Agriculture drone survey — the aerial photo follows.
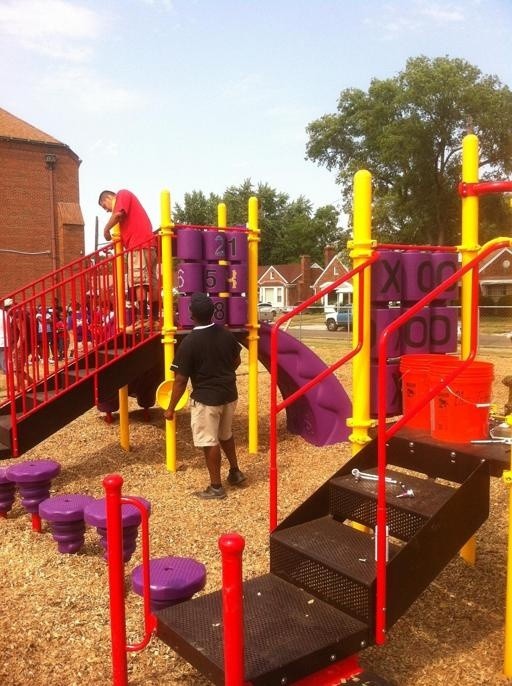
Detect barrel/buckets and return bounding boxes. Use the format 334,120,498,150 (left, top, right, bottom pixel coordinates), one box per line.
399,354,458,431
430,360,492,443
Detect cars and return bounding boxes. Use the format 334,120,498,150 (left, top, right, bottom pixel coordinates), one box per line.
257,303,280,323
324,303,353,332
281,301,315,314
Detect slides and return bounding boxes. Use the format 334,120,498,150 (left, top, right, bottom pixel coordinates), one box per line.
242,320,352,446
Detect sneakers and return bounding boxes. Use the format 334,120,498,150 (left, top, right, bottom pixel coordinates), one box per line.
126,318,159,333
197,486,226,500
227,470,247,487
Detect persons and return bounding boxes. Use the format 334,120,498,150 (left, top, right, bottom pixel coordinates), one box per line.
98,189,159,335
164,292,247,500
0,298,71,375
